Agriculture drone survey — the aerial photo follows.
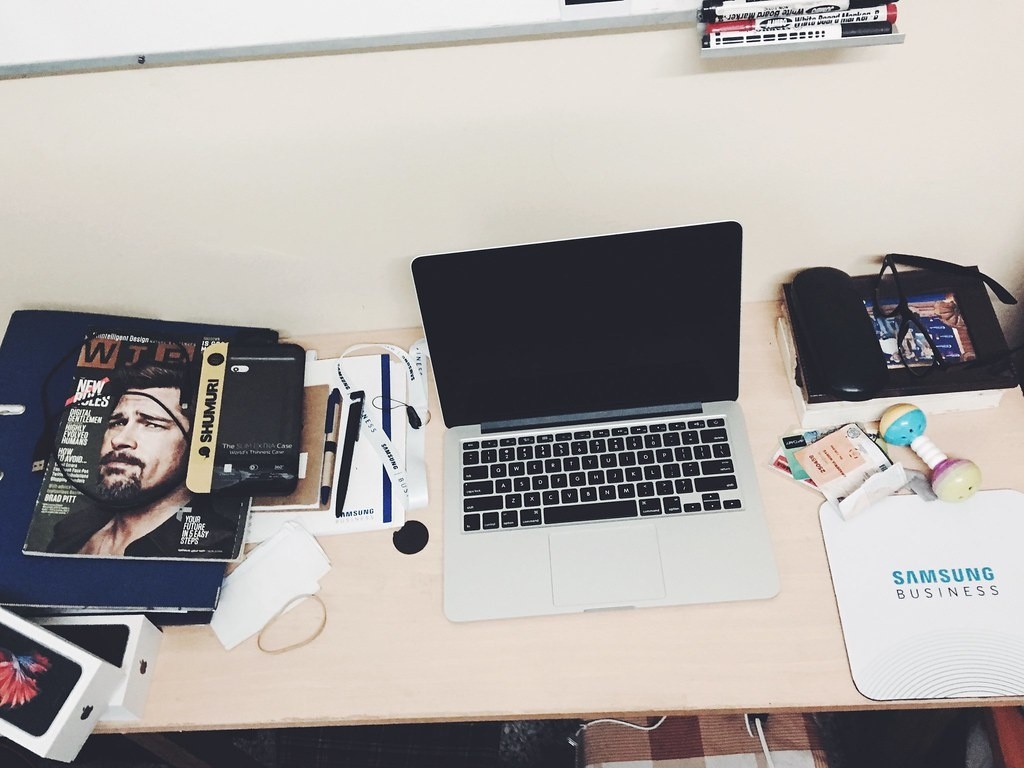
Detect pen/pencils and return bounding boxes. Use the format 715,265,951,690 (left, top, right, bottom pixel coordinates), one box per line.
335,390,364,518
320,388,342,505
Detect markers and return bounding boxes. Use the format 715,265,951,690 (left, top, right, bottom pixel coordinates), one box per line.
696,0,898,48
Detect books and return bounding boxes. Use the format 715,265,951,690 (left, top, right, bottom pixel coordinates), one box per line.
696,0,905,48
22,326,254,561
249,384,333,512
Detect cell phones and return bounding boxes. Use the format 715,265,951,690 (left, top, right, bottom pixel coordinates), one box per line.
186,342,305,496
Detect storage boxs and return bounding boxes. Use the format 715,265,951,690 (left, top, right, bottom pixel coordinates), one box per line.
34,613,165,721
0,605,126,764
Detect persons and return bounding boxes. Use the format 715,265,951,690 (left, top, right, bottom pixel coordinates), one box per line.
46,367,239,557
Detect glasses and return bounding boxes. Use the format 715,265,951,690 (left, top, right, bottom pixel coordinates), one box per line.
875,254,1024,378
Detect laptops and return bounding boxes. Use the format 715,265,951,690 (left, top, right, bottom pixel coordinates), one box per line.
412,221,782,623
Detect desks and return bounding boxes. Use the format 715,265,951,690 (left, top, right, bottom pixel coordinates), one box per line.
0,0,1024,737
